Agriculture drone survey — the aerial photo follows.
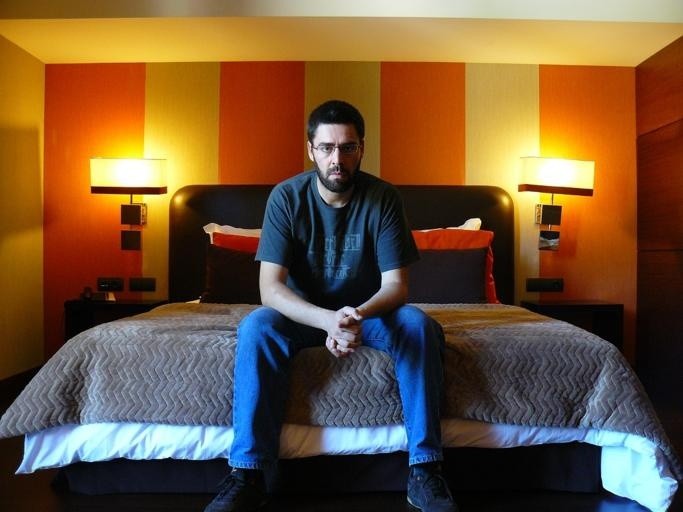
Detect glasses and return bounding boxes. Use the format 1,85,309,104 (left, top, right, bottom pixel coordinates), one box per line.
312,142,360,154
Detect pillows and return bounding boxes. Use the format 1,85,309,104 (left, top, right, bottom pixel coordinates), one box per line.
200,218,499,302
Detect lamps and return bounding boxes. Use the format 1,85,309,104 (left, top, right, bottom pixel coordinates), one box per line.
519,156,593,251
88,160,167,250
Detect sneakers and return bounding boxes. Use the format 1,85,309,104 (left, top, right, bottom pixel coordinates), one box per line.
204,470,267,512
407,471,457,511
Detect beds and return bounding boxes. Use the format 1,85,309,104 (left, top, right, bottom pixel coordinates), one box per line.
0,184,683,511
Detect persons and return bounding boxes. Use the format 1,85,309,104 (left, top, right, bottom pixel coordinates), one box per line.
198,98,460,512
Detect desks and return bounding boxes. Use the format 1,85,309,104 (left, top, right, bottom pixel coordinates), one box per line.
523,300,624,345
63,295,169,336
